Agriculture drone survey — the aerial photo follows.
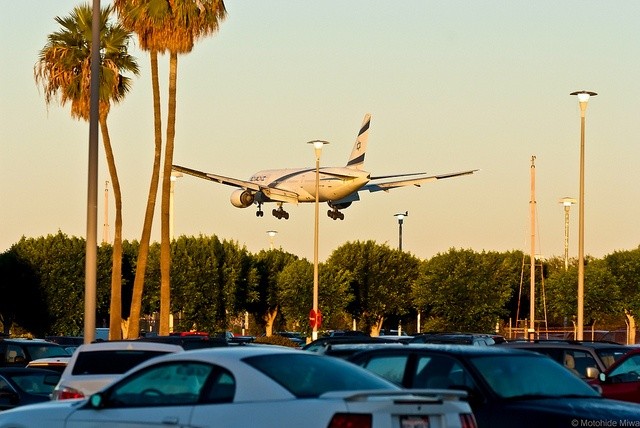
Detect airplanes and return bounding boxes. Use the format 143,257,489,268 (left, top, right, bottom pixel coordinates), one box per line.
172,113,480,220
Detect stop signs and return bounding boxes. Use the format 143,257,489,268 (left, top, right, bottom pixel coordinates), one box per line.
309,309,316,328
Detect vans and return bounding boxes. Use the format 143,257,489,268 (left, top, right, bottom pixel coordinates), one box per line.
490,344,640,394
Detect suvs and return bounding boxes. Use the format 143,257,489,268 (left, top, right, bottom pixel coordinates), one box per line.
0,338,69,367
43,342,184,401
326,344,640,428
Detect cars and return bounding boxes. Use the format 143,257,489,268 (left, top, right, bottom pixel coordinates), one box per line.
0,367,60,410
0,346,478,428
586,348,640,403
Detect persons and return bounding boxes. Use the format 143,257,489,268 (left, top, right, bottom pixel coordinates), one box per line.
140,333,146,339
305,332,312,345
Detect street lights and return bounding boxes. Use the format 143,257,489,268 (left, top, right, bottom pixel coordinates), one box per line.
570,91,598,341
308,140,329,341
560,197,576,272
394,212,408,251
